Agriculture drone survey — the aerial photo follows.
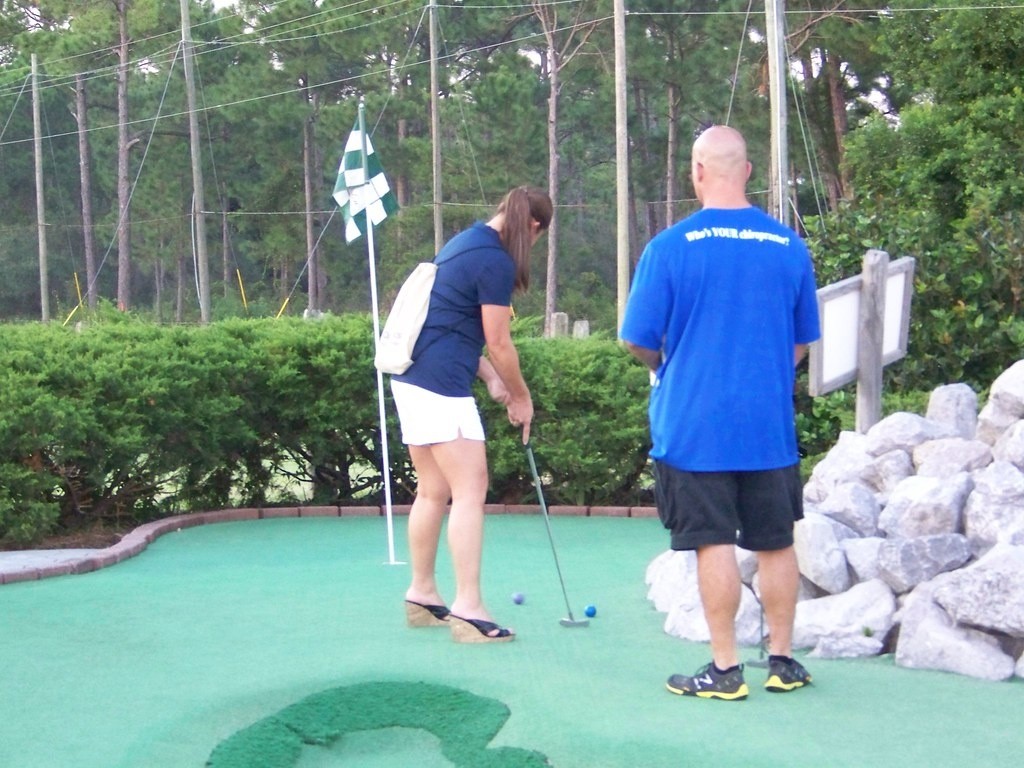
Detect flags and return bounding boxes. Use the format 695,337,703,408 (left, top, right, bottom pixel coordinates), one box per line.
333,102,398,246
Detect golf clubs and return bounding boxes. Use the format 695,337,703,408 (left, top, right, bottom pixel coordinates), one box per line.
518,423,591,630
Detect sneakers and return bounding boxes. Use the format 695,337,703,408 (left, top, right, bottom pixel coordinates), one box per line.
765,655,812,691
667,661,751,700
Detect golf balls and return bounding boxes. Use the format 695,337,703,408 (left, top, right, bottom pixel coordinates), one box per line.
584,604,597,618
511,592,525,606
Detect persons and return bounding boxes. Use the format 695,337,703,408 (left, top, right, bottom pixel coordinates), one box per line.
620,125,821,699
391,184,553,646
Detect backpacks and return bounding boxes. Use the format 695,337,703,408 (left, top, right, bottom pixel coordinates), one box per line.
374,246,513,375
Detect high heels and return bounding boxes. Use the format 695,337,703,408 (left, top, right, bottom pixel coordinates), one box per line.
449,613,516,644
406,600,450,627
